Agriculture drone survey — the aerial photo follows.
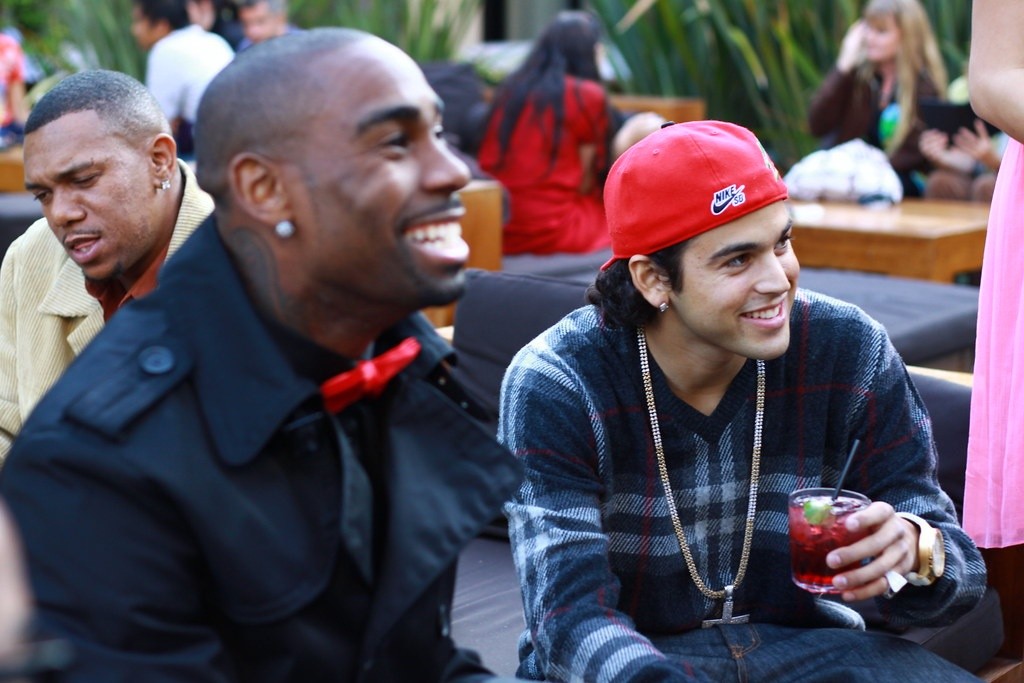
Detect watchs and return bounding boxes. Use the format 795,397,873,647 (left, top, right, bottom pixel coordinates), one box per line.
897,511,945,586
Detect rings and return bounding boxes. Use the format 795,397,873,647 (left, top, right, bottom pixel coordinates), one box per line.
883,572,909,599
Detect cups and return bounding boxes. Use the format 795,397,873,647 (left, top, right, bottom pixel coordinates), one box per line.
789,489,874,593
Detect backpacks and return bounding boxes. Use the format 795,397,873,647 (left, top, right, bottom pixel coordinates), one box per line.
420,58,496,153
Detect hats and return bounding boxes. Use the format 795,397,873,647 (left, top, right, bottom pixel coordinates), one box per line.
600,122,789,270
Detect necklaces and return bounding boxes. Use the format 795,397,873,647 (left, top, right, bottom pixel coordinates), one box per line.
637,331,763,625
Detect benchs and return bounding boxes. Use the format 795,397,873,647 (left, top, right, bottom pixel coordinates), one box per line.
452,252,980,543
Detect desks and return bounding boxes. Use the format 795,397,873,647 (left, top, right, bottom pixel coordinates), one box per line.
790,200,991,283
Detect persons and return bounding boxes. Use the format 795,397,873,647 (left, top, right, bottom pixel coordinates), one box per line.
1,0,1009,259
1,67,221,458
963,2,1023,551
498,119,990,683
1,30,524,683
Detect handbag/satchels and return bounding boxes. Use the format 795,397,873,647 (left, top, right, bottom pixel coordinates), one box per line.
782,140,899,204
